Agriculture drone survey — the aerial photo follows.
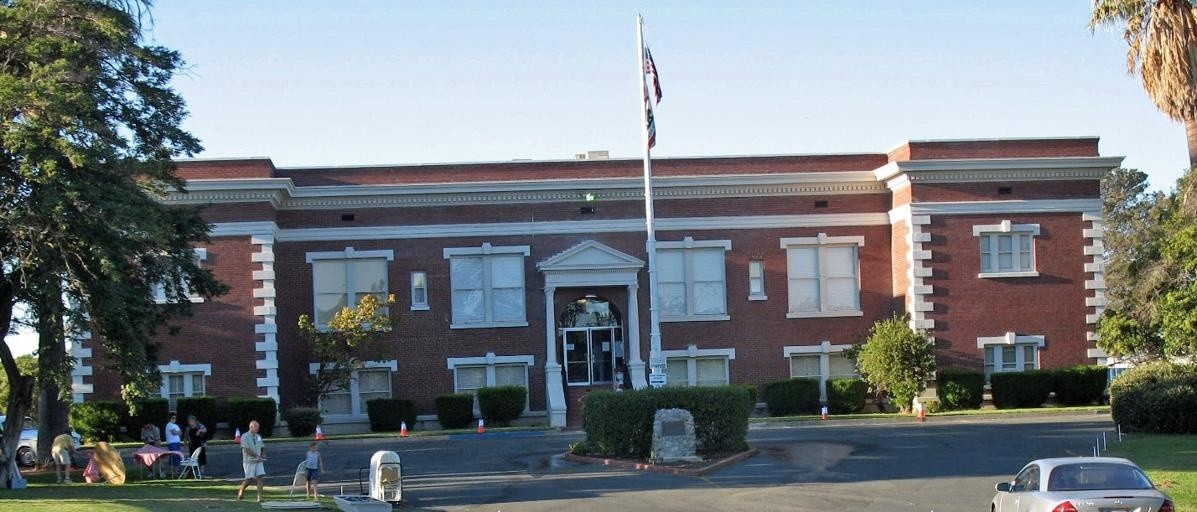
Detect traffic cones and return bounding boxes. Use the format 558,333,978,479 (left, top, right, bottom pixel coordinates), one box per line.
917,403,926,418
822,405,827,419
234,427,242,443
399,421,409,437
315,425,325,439
477,418,486,433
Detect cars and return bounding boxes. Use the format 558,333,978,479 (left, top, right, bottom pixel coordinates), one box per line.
989,457,1175,512
0,414,82,468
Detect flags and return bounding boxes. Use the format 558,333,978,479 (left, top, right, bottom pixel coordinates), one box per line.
645,29,664,150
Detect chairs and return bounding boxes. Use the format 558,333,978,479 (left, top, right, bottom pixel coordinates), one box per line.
177,445,204,480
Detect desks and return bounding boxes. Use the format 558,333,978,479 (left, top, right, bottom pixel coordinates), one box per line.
133,451,179,480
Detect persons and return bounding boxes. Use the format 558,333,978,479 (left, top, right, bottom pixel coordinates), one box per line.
165,411,208,475
238,420,267,502
50,429,81,483
139,418,164,480
304,440,325,501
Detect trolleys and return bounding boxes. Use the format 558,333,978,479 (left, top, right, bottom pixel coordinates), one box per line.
359,462,403,508
